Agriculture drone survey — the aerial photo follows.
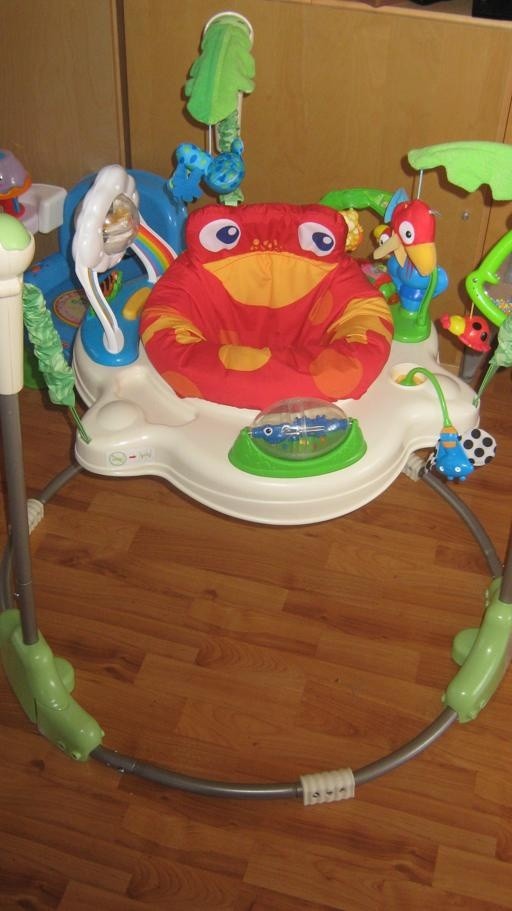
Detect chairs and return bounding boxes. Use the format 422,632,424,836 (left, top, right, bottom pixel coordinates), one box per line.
21,169,184,362
